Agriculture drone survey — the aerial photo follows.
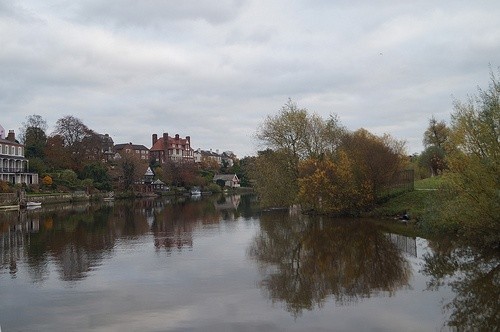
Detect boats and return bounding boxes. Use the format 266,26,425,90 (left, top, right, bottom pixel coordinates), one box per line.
21,200,41,206
190,189,202,195
